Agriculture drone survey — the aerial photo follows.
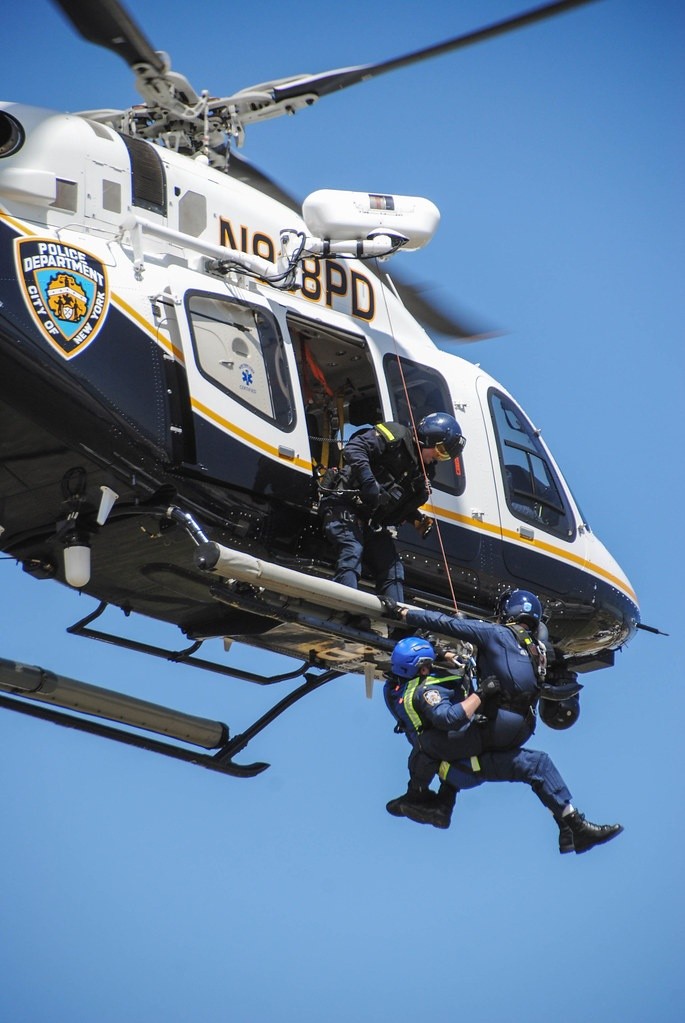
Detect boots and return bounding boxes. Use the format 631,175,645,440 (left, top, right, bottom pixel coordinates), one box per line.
400,786,459,829
554,817,575,853
385,777,430,816
564,809,624,853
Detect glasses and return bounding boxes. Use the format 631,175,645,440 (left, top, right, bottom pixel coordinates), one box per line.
435,442,452,462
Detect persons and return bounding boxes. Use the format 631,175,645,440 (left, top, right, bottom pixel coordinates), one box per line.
381,636,627,856
385,588,551,830
318,412,467,636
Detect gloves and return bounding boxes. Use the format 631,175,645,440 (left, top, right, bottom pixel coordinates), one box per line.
434,646,452,662
359,476,379,508
474,674,501,704
377,594,408,621
413,513,433,538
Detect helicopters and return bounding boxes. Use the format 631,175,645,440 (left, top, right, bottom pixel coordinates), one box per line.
0,1,673,783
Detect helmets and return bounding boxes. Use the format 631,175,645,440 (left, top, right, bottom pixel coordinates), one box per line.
390,637,436,679
414,412,466,461
502,589,541,626
